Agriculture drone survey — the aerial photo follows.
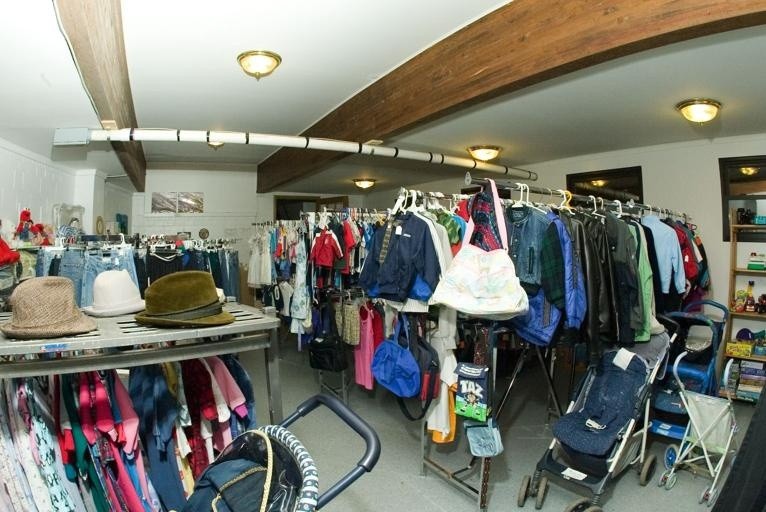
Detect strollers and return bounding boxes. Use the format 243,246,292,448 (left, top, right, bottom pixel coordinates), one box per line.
162,387,381,512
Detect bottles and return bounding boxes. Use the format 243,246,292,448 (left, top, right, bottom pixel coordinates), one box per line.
748,294,754,306
736,207,765,225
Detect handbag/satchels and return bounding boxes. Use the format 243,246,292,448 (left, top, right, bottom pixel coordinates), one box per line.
462,416,507,459
368,312,421,403
387,315,446,423
305,301,346,372
424,241,536,321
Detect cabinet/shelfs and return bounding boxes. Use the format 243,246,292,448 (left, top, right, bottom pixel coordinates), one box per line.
717,209,766,404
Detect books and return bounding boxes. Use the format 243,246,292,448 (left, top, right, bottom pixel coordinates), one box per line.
720,363,766,403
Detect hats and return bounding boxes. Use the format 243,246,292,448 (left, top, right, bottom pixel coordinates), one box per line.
0,274,101,341
131,267,237,328
83,266,148,318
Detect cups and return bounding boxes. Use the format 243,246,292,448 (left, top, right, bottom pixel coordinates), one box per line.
755,345,766,355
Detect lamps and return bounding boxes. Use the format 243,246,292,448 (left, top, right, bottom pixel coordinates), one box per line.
351,178,377,193
237,49,282,81
465,144,503,163
674,97,723,126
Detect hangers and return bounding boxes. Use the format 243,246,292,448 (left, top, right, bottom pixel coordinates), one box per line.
254,220,301,231
514,182,687,222
114,232,132,250
394,188,470,215
143,235,175,249
42,237,68,250
194,238,232,250
295,209,382,223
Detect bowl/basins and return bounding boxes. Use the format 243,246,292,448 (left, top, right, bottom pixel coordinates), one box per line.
748,263,765,269
